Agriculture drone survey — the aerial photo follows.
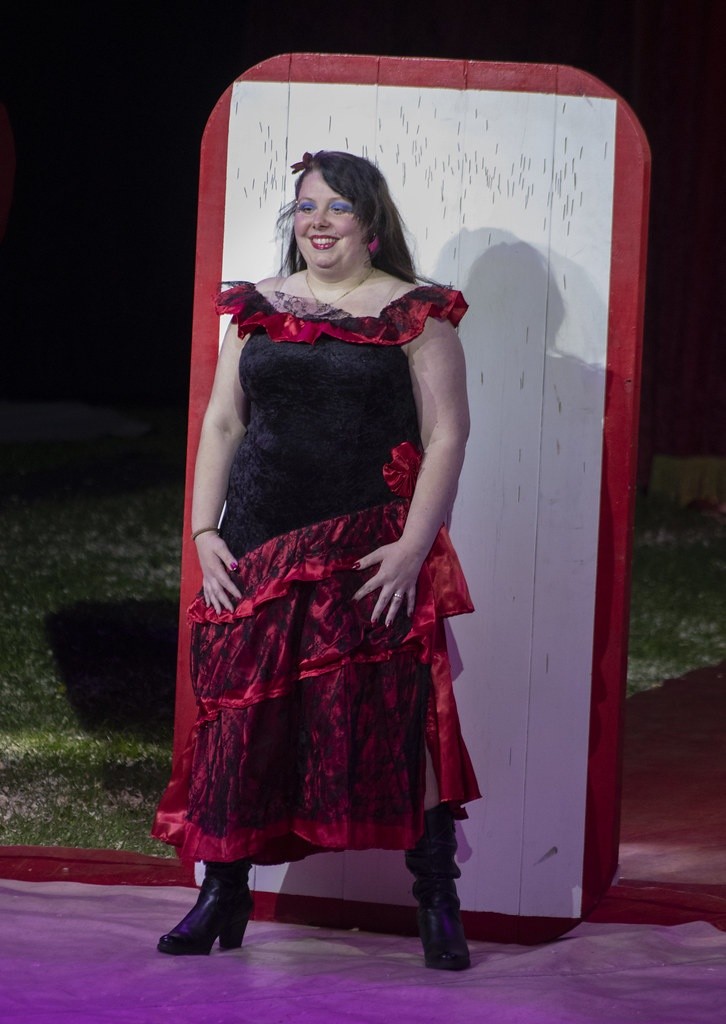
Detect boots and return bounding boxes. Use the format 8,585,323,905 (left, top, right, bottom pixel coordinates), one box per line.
404,802,471,971
156,859,254,956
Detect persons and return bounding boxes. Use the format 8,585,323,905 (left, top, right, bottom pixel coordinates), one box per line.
157,150,483,969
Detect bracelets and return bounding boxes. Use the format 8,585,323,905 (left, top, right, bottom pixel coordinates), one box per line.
191,528,219,540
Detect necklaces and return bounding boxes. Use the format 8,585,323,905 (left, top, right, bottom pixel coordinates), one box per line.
305,269,374,311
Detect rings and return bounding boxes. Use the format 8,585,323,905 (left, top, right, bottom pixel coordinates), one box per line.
394,593,403,599
